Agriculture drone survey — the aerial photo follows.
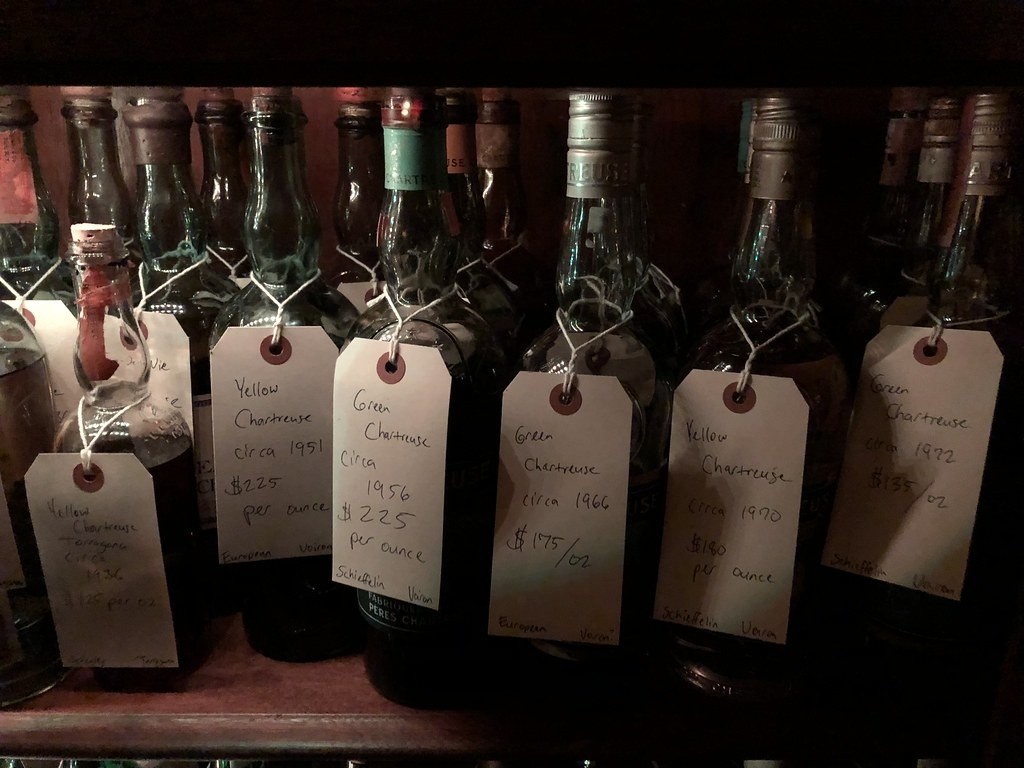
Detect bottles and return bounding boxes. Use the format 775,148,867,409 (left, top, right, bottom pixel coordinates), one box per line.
0,84,1024,768
